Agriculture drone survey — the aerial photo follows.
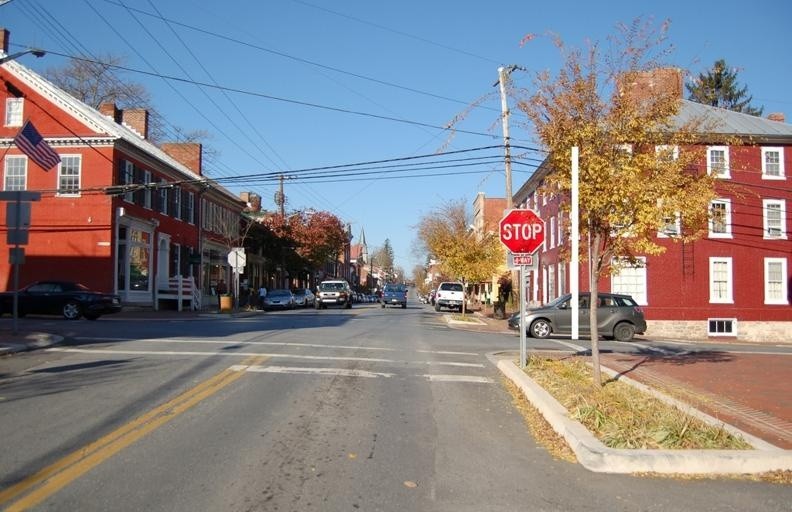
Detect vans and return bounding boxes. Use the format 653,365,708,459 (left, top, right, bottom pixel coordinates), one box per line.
507,291,647,342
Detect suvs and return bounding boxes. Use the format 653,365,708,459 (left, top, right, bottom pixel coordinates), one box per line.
315,279,353,310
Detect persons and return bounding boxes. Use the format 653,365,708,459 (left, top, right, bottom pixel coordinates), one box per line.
214,278,267,312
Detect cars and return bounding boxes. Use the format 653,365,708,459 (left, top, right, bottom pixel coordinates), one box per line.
416,289,436,306
0,278,123,321
263,288,316,312
352,290,381,303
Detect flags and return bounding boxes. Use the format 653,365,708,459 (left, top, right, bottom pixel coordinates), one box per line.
14,120,62,172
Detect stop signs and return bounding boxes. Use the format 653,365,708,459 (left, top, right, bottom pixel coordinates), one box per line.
498,209,546,255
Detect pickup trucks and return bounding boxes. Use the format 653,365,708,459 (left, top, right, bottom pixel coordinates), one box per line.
434,281,467,313
380,283,408,309
